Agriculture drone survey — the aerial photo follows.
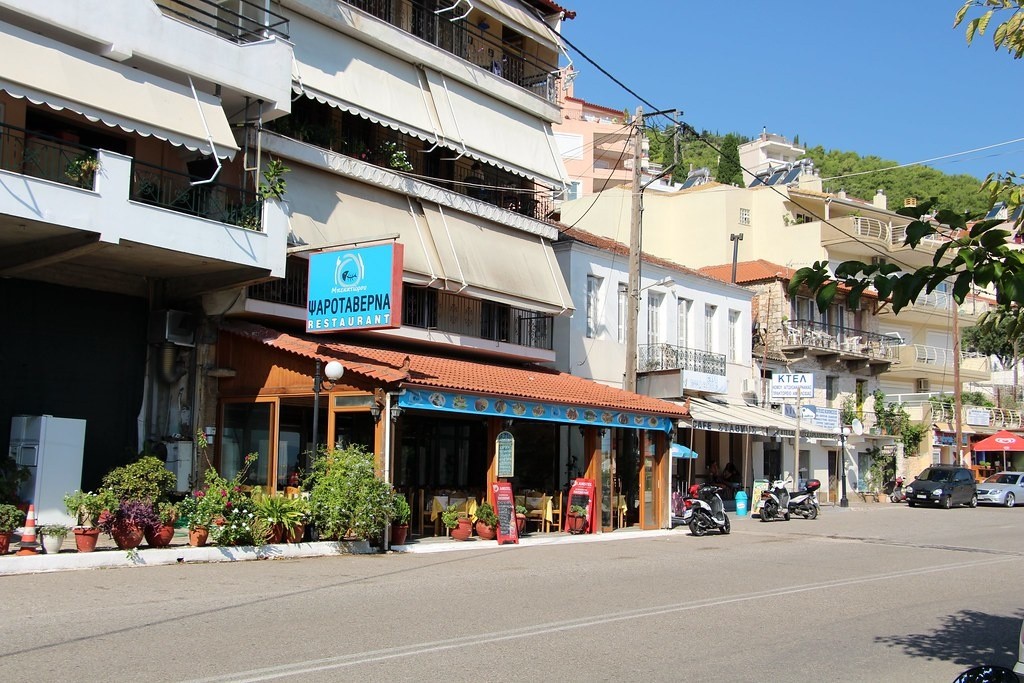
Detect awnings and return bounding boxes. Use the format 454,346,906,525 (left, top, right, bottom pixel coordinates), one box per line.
664,395,844,441
251,150,576,318
269,1,572,193
0,20,242,164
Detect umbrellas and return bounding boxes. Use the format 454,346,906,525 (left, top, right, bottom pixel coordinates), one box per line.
649,440,698,458
970,428,1024,472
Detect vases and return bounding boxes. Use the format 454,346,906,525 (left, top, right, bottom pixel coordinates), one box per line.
341,528,357,540
218,522,241,545
113,519,144,549
72,527,100,552
189,524,208,547
145,522,175,547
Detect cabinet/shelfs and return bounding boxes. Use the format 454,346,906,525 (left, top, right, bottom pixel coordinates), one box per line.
163,440,194,496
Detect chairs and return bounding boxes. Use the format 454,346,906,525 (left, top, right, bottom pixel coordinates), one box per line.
419,489,439,537
784,325,875,357
442,492,485,537
528,491,562,533
405,487,413,539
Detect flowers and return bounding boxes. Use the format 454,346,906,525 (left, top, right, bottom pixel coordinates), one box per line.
64,455,177,564
64,153,103,183
357,139,414,172
295,437,397,541
175,428,259,546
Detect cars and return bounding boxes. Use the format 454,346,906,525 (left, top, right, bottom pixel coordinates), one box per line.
976,471,1024,508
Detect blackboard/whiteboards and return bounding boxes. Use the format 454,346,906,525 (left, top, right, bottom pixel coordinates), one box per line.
496,432,515,477
567,477,596,530
492,482,518,540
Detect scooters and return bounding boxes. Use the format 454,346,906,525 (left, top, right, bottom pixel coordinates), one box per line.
682,483,732,536
756,472,794,522
890,476,906,503
788,479,821,520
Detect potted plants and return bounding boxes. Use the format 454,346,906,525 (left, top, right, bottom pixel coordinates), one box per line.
862,446,894,504
515,506,527,531
873,389,911,435
39,523,72,554
979,461,985,469
442,504,472,542
0,503,26,555
986,462,991,469
392,493,410,544
251,487,308,544
568,505,587,531
837,392,856,434
476,502,499,539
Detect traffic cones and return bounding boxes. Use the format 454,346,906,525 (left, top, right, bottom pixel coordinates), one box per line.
15,504,41,557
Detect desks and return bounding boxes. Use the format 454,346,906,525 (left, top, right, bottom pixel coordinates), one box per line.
498,191,520,212
426,496,553,537
603,495,627,528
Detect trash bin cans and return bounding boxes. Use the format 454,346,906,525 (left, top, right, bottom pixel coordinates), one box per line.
735,490,748,515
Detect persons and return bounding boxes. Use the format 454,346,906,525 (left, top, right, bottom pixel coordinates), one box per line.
708,460,742,500
562,455,583,493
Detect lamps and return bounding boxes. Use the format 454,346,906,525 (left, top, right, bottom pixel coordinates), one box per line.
370,401,382,422
597,427,608,437
668,428,676,441
390,402,402,423
478,17,490,30
579,424,585,438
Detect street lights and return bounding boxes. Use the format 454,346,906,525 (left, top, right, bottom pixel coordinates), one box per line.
622,275,677,520
729,233,744,284
835,425,852,507
307,357,345,488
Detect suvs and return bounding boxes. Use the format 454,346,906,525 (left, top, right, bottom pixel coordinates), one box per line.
904,463,978,509
